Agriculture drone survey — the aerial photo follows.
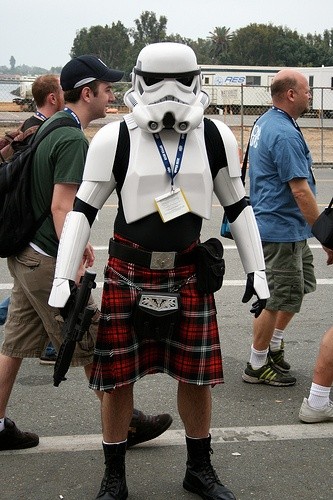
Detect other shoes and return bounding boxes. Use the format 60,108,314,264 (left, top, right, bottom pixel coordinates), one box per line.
40,354,57,365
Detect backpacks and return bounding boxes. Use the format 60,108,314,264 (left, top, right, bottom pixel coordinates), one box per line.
0,117,81,259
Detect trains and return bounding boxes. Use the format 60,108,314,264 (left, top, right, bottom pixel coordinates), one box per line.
197,65,333,118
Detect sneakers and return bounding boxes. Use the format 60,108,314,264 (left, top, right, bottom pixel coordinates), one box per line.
299,397,333,423
0,417,39,451
241,358,298,386
126,409,173,450
268,339,291,372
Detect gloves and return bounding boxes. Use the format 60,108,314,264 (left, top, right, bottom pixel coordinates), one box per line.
61,281,80,322
242,274,266,319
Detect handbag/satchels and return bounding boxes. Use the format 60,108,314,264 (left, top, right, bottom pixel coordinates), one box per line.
220,211,233,241
311,208,333,247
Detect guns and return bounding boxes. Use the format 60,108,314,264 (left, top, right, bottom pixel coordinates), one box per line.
53,266,99,389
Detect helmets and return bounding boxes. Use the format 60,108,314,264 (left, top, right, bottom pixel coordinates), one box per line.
123,42,212,133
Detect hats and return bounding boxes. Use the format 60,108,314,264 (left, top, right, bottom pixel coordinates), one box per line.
60,55,125,91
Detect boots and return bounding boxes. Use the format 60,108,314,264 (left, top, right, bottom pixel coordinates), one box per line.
94,438,128,500
182,433,236,500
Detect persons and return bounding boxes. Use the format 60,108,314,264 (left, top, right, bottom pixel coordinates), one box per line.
46,42,270,500
241,69,333,387
298,246,333,423
0,55,172,451
